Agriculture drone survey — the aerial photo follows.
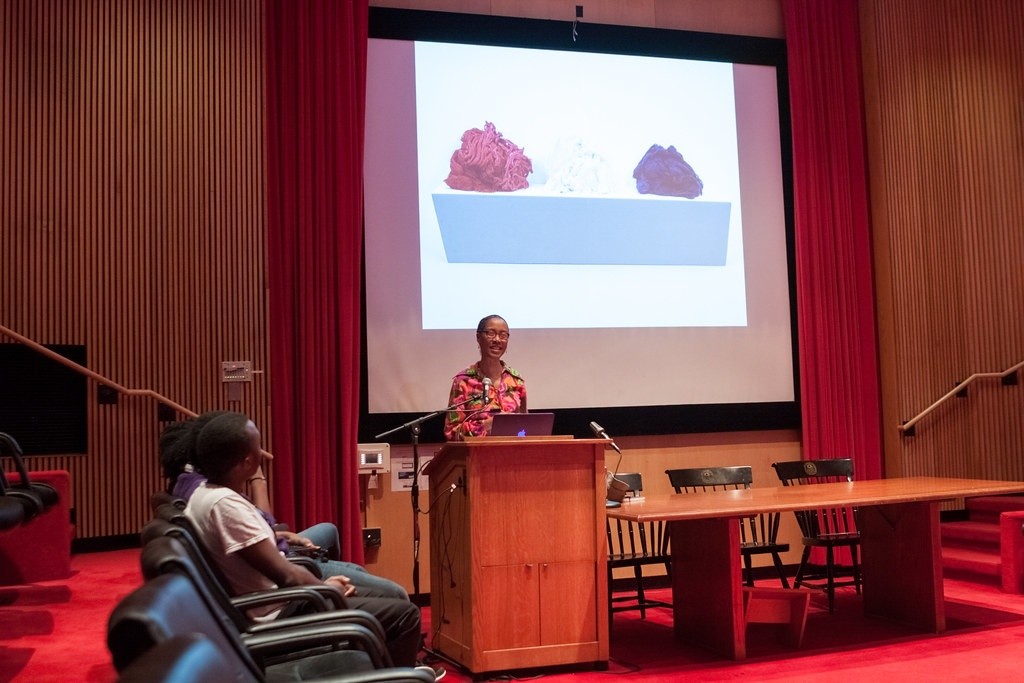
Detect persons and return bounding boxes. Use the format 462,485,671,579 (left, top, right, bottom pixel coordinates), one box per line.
443,314,528,442
157,408,446,683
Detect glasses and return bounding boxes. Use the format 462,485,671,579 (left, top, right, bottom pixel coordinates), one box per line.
477,328,509,339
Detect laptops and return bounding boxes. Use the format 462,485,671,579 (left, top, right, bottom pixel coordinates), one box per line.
491,413,555,437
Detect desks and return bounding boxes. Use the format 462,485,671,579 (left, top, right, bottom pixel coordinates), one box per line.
606,476,1024,661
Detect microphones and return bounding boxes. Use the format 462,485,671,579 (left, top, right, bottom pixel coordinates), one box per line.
482,377,492,404
590,421,621,454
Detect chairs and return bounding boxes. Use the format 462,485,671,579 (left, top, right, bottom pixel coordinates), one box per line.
665,465,791,588
604,472,674,630
0,429,61,534
104,491,444,683
772,458,863,613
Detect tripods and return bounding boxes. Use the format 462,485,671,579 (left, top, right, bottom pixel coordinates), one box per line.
376,394,469,675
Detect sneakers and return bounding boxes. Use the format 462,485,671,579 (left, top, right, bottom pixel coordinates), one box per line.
415,662,446,681
